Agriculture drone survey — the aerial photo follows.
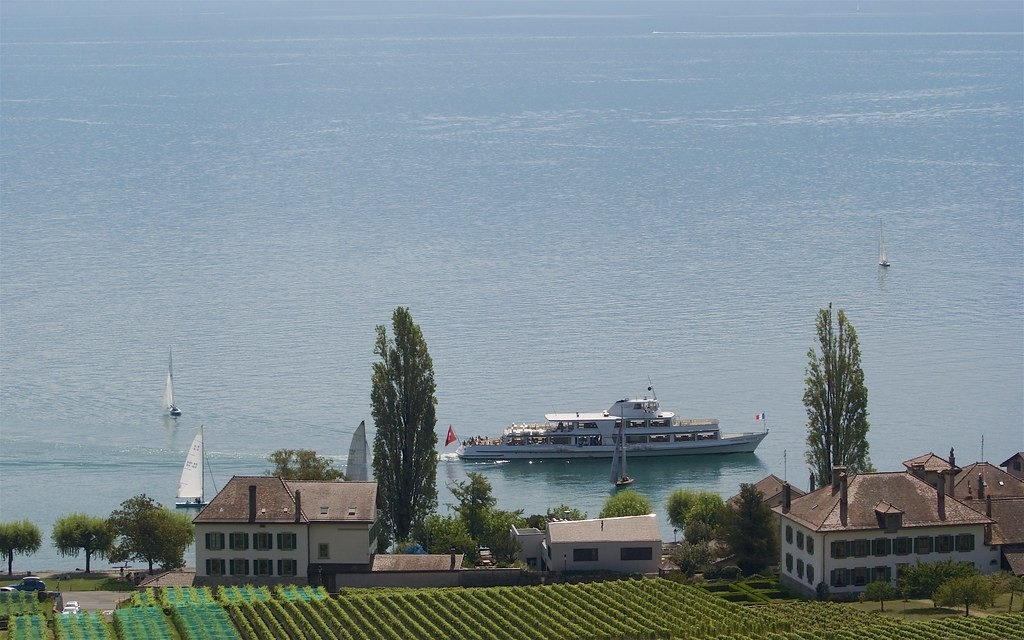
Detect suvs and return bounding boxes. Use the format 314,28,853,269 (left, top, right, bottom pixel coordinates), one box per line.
8,577,46,590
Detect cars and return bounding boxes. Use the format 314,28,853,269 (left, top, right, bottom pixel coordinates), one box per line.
62,601,81,615
0,586,19,593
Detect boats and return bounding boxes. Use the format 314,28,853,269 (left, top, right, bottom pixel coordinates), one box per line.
455,375,771,461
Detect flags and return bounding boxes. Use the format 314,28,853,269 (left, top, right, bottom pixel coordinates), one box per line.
444,425,456,447
756,412,765,420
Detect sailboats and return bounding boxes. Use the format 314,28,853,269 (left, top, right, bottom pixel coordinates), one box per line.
611,422,635,487
163,347,181,415
174,424,213,509
878,215,891,266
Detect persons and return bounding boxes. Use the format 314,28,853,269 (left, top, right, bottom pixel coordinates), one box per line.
620,472,630,482
119,566,142,585
